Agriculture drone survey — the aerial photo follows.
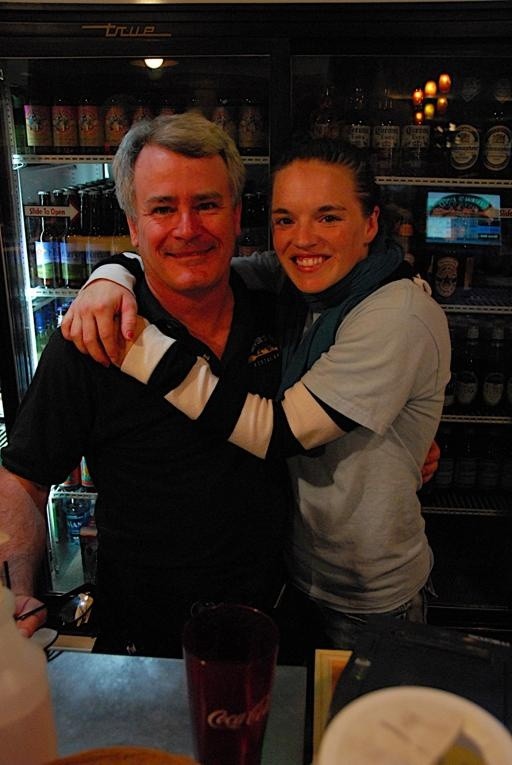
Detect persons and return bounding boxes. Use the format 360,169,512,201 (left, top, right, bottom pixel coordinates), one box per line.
54,134,455,699
0,106,442,667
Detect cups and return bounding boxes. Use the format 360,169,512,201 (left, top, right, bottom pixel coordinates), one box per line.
180,605,279,765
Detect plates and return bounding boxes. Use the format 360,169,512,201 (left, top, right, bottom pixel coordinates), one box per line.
314,686,512,765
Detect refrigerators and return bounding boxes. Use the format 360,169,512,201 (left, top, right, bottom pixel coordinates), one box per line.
0,0,511,635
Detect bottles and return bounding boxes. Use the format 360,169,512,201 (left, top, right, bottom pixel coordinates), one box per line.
302,70,432,174
233,187,269,255
17,63,267,158
2,589,59,763
34,178,139,293
446,62,509,181
422,422,504,498
441,313,510,419
57,453,95,493
36,307,68,353
386,188,509,304
62,493,91,546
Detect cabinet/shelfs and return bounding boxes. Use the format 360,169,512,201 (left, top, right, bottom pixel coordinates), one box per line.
2,98,512,640
